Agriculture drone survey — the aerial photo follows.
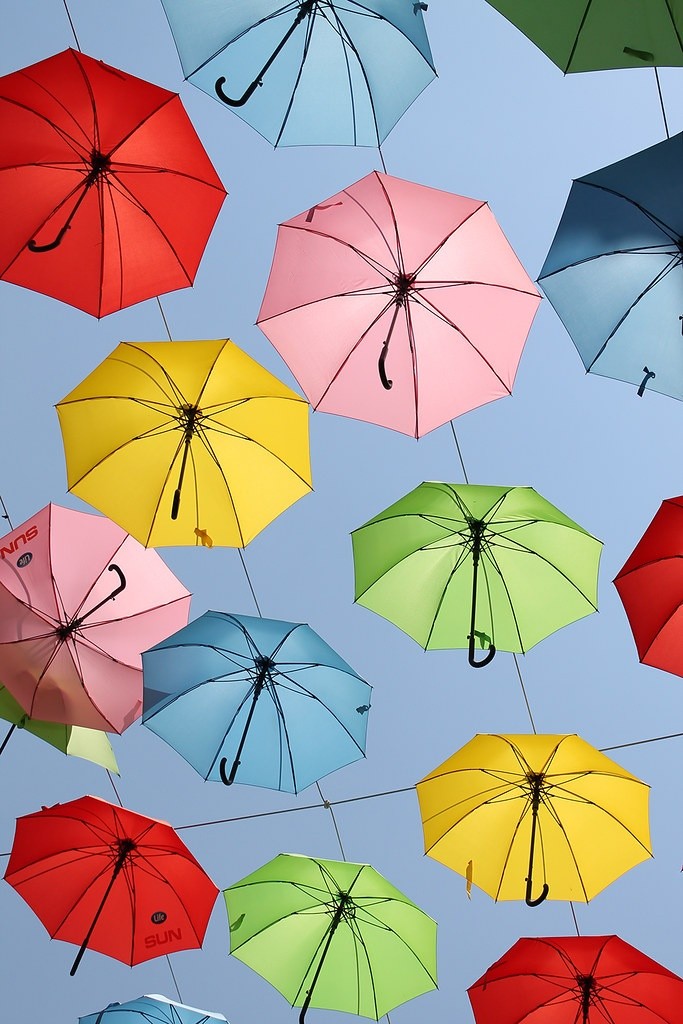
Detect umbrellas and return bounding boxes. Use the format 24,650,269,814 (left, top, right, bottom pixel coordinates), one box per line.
348,479,606,670
0,793,224,977
415,732,658,908
466,933,683,1024
252,169,546,443
533,130,683,401
0,499,192,780
138,608,376,798
0,45,231,320
75,990,233,1024
221,851,440,1024
157,0,440,151
613,492,683,678
484,0,682,78
53,337,316,551
